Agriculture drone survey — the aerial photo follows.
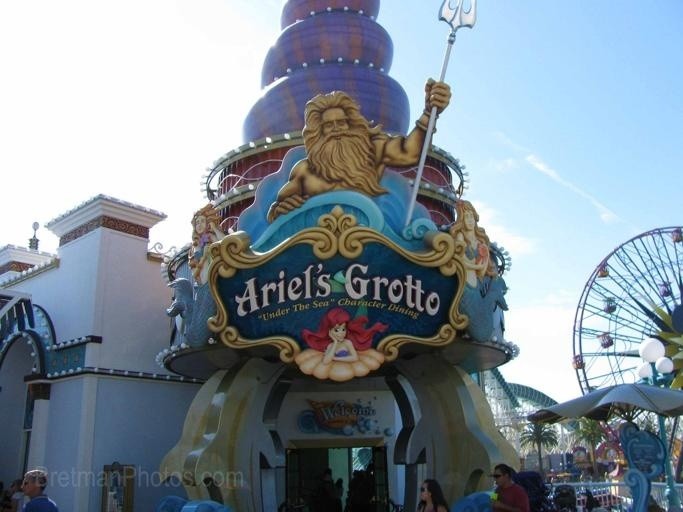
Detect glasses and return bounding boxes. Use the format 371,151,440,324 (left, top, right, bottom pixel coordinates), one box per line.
494,475,500,477
421,488,425,492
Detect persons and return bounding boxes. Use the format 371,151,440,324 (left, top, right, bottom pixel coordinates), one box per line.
417,479,448,512
491,464,530,512
310,468,374,512
266,80,451,224
450,200,491,287
302,308,388,364
0,470,58,512
187,205,228,284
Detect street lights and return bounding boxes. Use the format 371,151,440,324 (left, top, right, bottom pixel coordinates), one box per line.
637,338,682,511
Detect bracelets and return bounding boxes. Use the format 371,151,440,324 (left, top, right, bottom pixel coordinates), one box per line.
414,121,436,135
422,110,439,119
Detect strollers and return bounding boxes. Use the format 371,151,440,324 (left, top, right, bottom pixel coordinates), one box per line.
514,471,553,512
553,484,577,511
